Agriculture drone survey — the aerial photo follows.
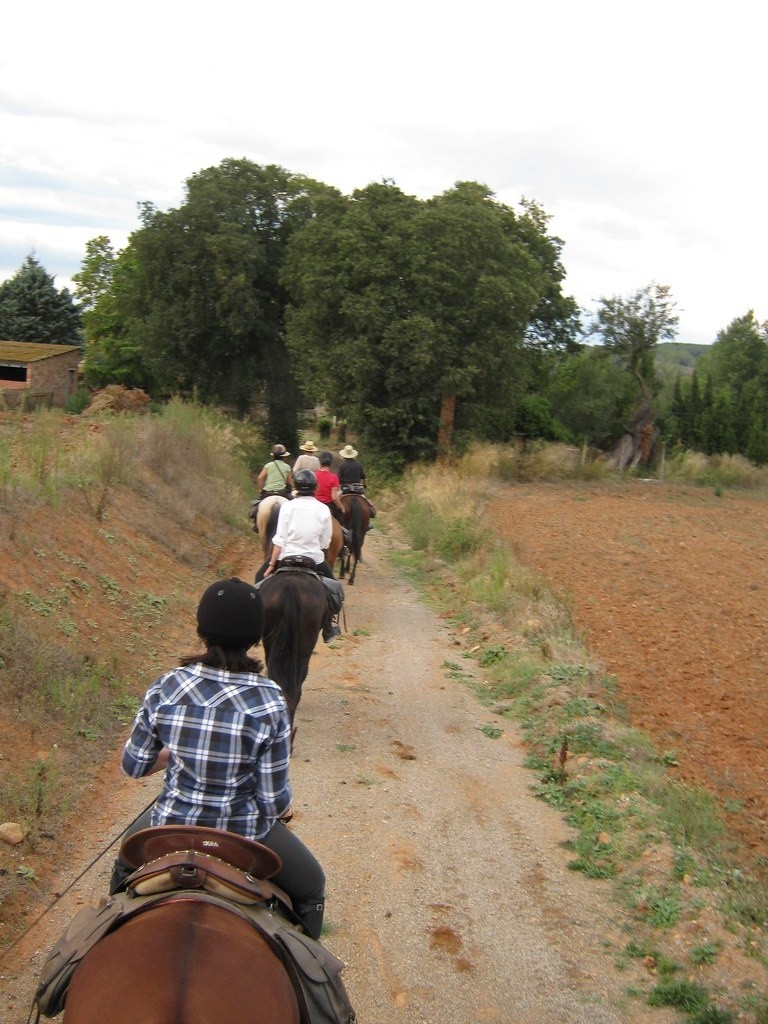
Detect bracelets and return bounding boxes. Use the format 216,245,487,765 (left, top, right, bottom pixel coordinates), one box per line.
269,562,275,566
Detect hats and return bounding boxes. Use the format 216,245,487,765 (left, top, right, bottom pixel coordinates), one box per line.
270,444,291,456
339,445,359,458
299,441,318,452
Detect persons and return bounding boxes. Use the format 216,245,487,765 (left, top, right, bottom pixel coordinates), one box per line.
109,578,326,942
255,470,340,644
252,440,367,553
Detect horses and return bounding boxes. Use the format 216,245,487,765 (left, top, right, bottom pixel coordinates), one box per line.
61,653,308,1024
316,515,344,574
339,496,370,586
256,495,289,561
259,569,326,731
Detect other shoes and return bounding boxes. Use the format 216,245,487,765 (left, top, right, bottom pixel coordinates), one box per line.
368,523,373,529
323,626,340,643
253,527,259,533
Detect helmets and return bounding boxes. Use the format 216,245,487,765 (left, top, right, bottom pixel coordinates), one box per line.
320,451,333,464
294,469,317,491
196,577,261,644
